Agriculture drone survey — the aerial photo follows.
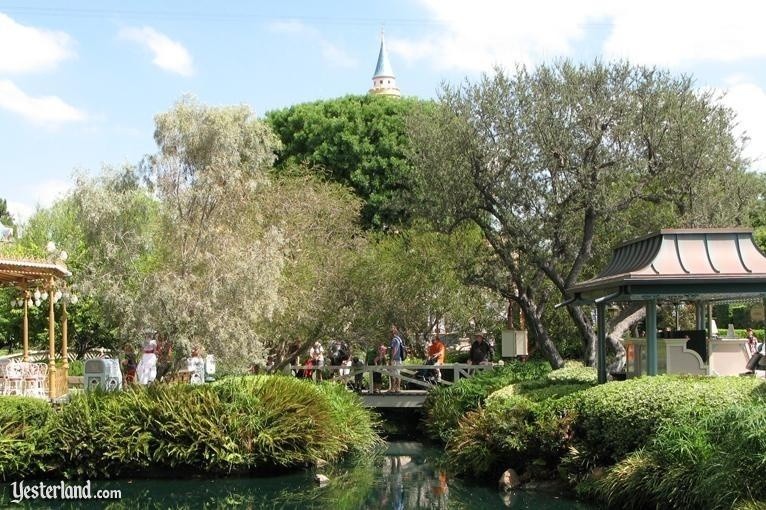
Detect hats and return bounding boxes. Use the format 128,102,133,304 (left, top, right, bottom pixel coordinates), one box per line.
473,332,483,337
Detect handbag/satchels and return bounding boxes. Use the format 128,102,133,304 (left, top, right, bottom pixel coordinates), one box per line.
330,357,342,366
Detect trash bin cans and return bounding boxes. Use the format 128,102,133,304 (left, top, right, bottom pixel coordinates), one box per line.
84,359,122,393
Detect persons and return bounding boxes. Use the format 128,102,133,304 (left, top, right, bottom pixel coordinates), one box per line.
415,336,445,385
120,325,403,395
640,325,691,349
486,338,495,366
745,328,759,355
359,453,451,510
467,332,489,375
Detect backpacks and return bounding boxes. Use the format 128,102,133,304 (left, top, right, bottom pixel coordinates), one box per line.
336,343,350,360
393,337,407,361
